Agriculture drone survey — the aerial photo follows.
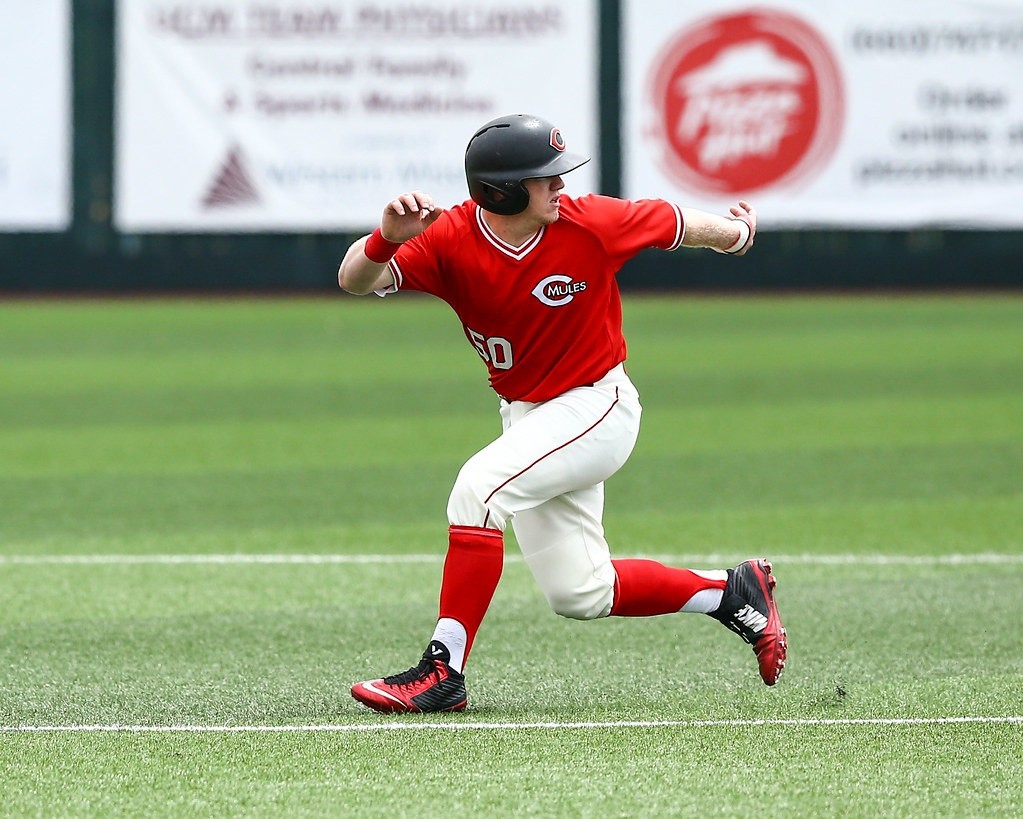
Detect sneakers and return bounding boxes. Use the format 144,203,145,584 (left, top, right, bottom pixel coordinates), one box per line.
705,558,787,686
350,640,468,714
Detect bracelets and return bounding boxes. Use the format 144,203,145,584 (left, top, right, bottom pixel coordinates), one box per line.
722,218,751,254
364,226,405,265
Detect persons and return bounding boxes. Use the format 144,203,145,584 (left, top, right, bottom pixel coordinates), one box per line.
337,113,786,713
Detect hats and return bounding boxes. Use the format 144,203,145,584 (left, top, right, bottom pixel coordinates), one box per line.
464,114,591,215
